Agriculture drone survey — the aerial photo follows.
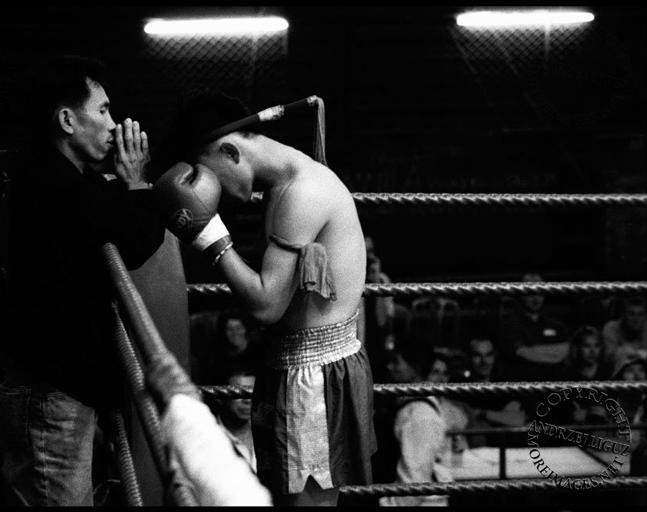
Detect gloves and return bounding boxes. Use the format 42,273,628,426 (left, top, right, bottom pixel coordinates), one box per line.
154,161,233,271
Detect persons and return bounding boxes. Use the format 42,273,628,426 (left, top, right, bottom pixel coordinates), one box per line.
180,236,647,507
1,59,167,509
154,97,376,509
